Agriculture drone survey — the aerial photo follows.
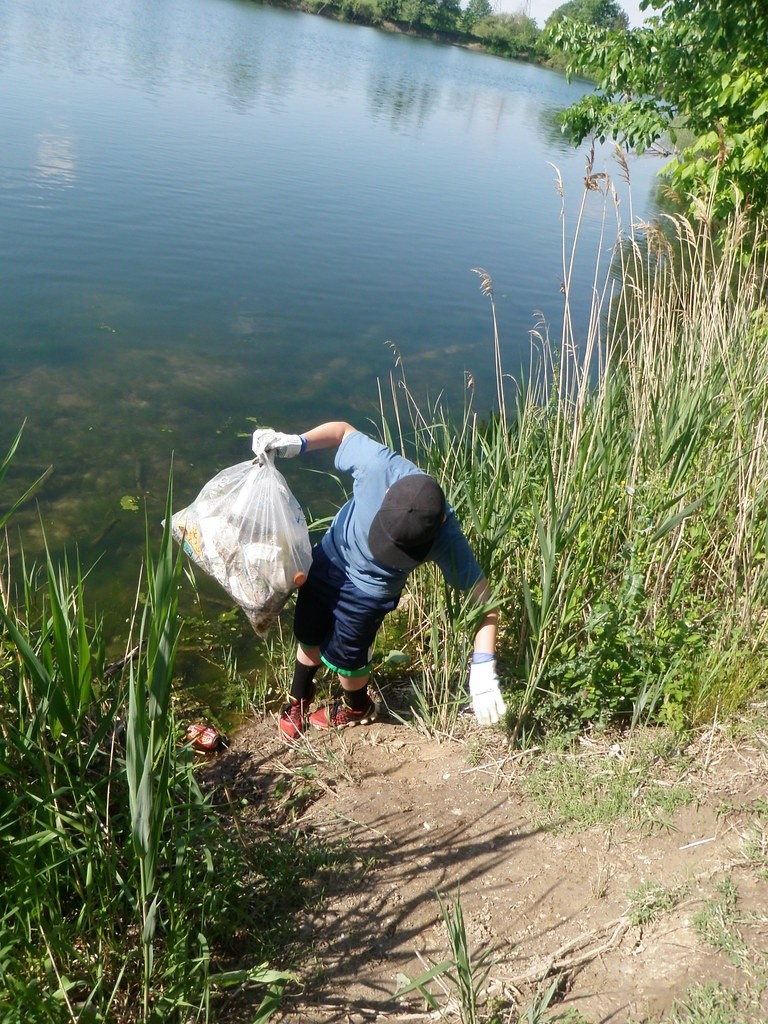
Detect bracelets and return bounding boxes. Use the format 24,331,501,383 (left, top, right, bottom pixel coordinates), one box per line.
299,435,307,456
472,653,495,663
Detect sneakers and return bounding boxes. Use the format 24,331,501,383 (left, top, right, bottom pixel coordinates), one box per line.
309,695,379,730
278,679,318,741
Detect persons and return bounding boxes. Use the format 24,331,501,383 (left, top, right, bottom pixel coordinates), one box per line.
253,421,506,744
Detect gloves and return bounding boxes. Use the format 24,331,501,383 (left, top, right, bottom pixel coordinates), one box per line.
468,652,506,726
252,428,307,461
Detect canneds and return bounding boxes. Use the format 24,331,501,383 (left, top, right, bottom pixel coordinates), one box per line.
187,724,220,749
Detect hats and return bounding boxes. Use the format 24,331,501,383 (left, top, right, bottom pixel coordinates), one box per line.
368,474,446,571
